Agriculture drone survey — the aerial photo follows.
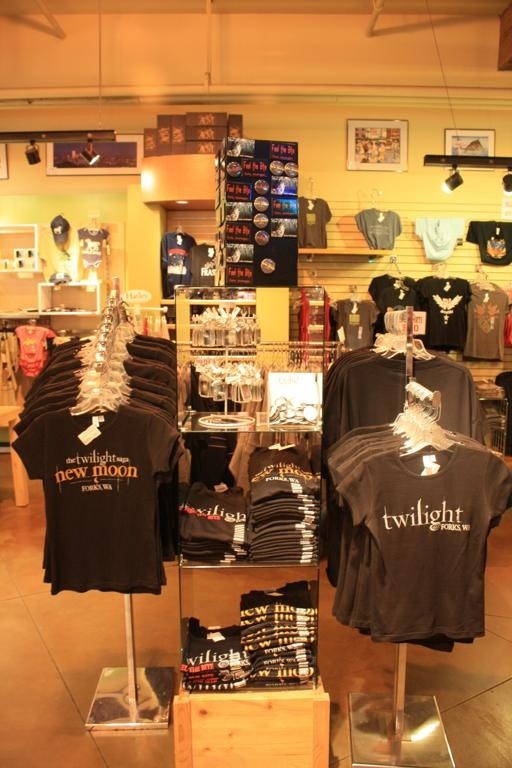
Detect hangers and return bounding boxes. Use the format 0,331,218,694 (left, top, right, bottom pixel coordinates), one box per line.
433,262,455,282
390,380,458,475
370,306,439,369
471,270,497,292
68,299,140,422
183,337,332,404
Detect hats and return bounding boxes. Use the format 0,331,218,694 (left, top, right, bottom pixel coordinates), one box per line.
50,273,71,285
51,216,70,242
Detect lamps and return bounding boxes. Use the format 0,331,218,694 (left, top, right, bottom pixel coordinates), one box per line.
79,143,101,165
502,169,512,194
25,147,41,165
441,167,463,193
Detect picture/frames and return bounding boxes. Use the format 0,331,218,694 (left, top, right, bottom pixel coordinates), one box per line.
46,135,145,177
346,118,409,173
444,128,496,156
